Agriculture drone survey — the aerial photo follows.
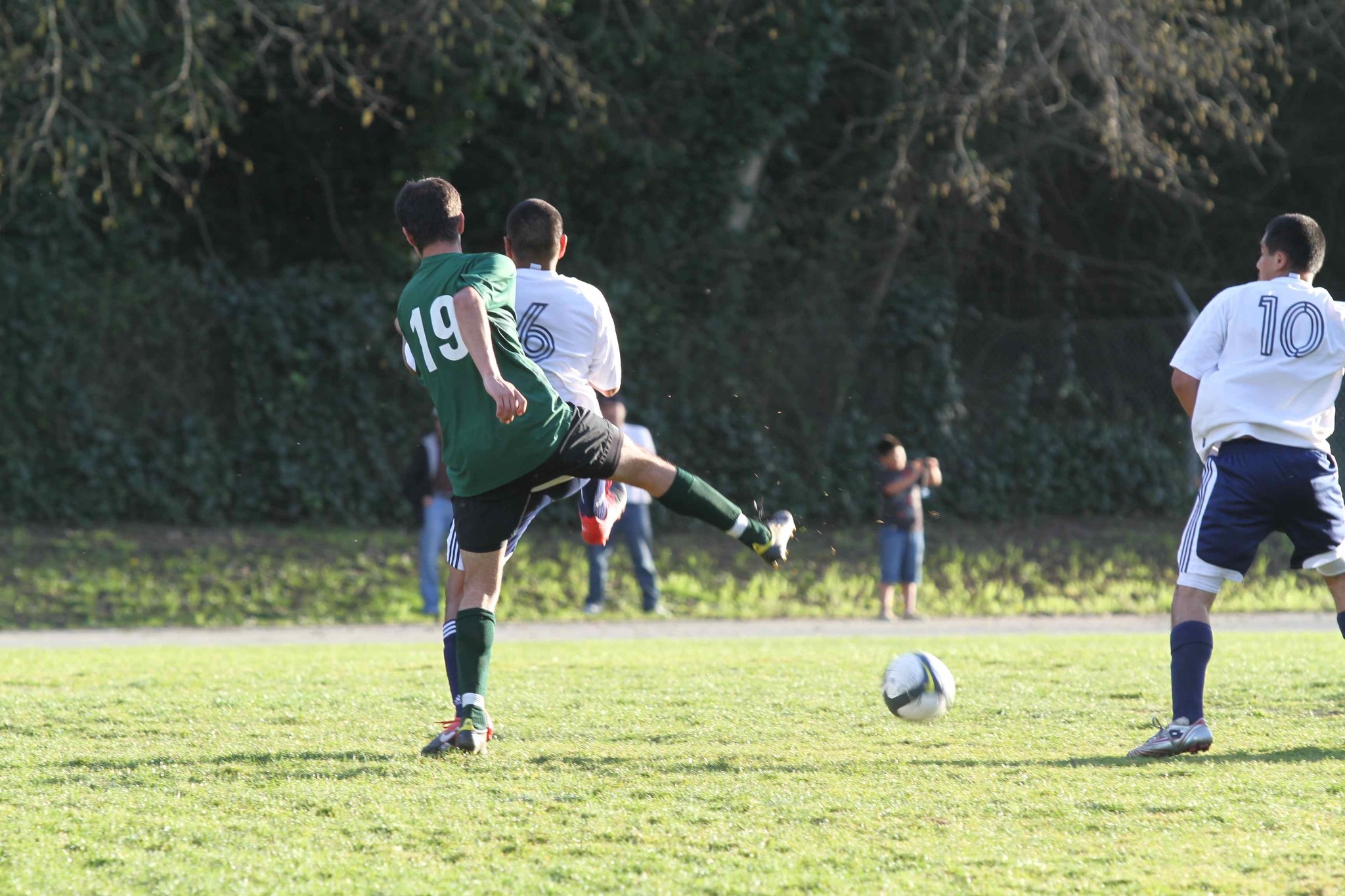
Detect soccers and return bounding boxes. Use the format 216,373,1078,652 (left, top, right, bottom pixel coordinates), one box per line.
882,651,958,725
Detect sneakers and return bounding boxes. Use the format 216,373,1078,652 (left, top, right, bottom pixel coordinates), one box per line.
751,510,796,568
579,481,628,545
454,719,488,755
1128,716,1212,758
420,719,493,757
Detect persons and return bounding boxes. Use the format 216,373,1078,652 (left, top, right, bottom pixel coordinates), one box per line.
1128,213,1345,758
443,199,629,740
396,176,796,753
414,411,451,614
581,395,667,616
877,433,943,623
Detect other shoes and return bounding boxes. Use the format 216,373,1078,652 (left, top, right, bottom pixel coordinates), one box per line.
880,611,897,621
582,604,600,613
904,612,928,620
644,606,668,618
